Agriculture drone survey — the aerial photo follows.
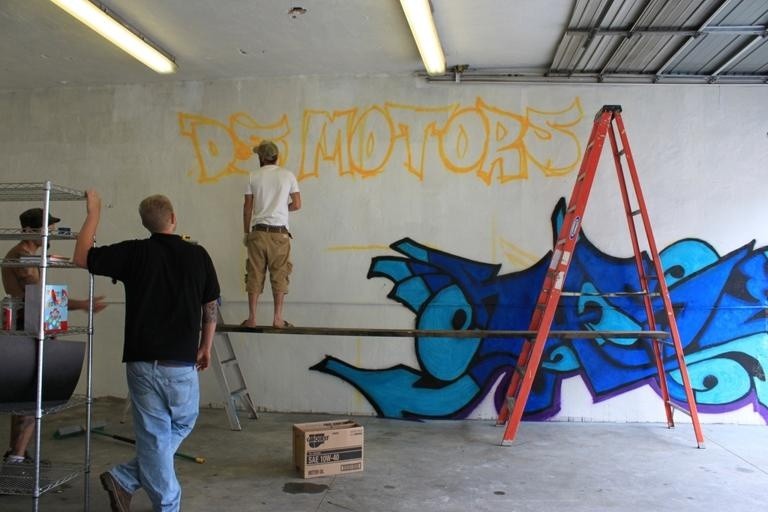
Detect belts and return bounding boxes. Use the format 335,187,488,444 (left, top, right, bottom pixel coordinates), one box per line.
252,223,288,234
152,361,192,367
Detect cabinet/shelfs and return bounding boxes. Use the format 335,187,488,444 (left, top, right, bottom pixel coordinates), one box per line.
2,180,100,511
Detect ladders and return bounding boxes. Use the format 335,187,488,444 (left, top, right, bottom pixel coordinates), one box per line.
495,104,706,447
211,310,258,431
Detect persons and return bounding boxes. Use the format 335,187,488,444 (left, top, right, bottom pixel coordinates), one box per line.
1,208,107,464
73,189,220,512
241,140,301,329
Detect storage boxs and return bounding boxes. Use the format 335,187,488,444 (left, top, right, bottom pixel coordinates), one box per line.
290,416,367,480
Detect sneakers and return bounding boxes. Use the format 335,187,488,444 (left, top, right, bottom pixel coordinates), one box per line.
101,472,133,512
4,449,47,463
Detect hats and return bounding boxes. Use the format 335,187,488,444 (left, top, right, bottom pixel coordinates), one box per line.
19,208,60,228
253,139,279,162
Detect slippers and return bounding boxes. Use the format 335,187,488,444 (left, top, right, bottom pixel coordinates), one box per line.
282,320,294,328
240,320,248,326
